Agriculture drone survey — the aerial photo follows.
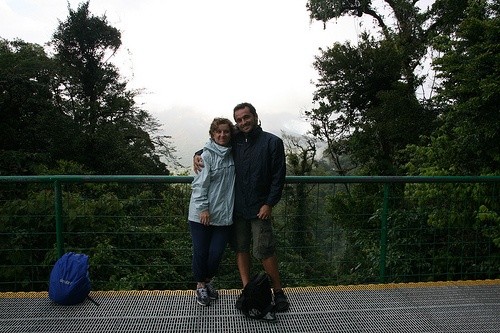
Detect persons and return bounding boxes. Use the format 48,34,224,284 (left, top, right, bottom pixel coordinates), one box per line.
194,103,291,313
188,118,236,306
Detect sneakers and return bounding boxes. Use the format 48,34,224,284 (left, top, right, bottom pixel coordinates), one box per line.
196,286,211,307
204,284,218,300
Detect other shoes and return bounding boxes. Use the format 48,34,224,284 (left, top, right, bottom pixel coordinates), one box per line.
273,288,291,313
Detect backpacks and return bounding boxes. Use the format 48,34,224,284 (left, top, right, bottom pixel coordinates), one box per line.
234,278,277,322
48,252,101,306
